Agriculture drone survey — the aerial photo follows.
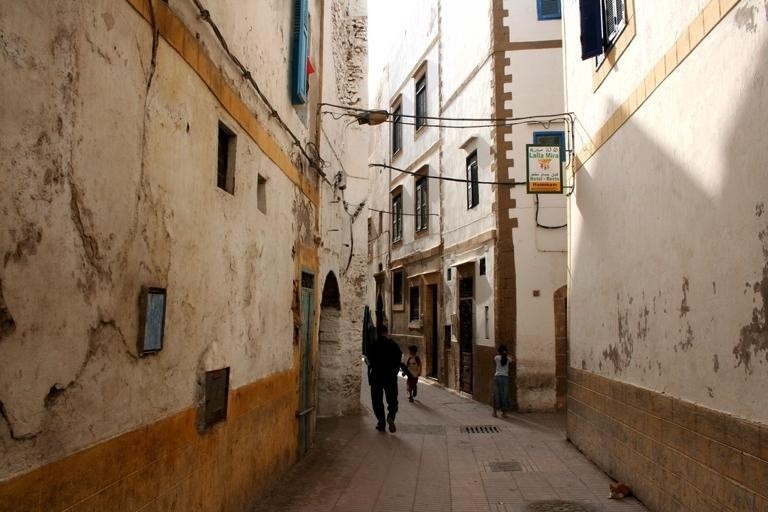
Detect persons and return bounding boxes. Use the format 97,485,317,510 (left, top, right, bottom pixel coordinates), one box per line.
367,326,401,433
402,345,422,402
491,344,514,417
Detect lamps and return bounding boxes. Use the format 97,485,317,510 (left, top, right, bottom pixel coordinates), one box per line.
344,109,389,132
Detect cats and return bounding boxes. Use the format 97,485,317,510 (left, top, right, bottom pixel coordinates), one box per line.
607,481,632,499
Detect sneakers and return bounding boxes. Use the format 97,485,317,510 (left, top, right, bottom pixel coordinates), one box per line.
388,414,396,432
376,425,385,431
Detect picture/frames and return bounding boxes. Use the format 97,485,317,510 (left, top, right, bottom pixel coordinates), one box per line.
134,284,166,356
